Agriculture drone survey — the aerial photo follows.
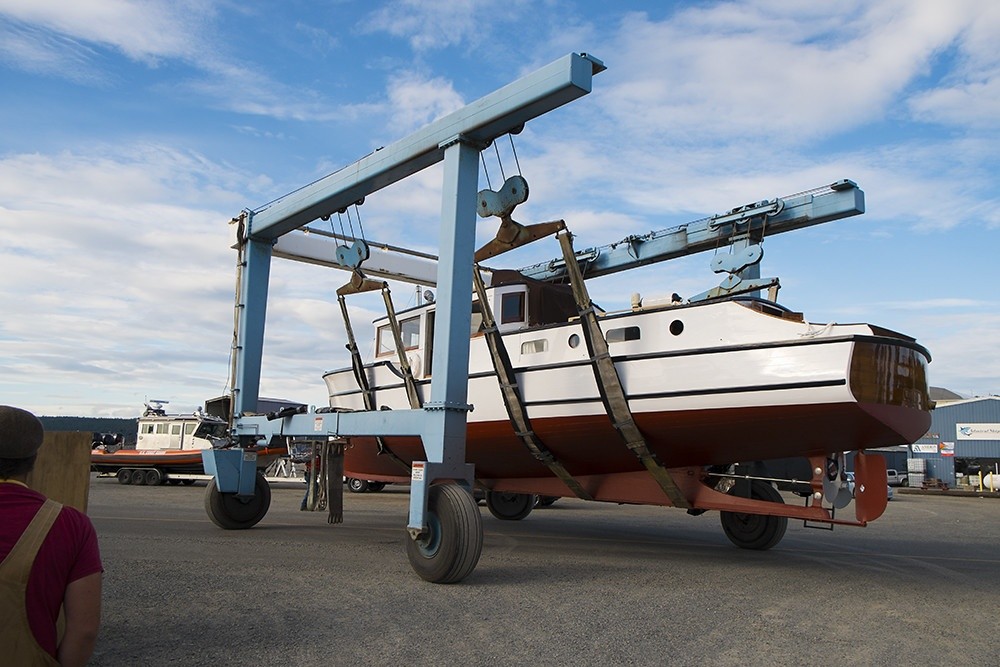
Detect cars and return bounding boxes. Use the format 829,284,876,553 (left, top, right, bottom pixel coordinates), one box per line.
844,471,893,501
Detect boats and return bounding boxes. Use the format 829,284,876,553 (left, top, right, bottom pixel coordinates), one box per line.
321,282,937,532
86,400,287,474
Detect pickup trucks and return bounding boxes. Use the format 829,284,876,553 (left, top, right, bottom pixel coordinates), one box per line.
886,469,909,487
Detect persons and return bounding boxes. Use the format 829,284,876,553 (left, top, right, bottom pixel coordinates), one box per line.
0,405,105,667
